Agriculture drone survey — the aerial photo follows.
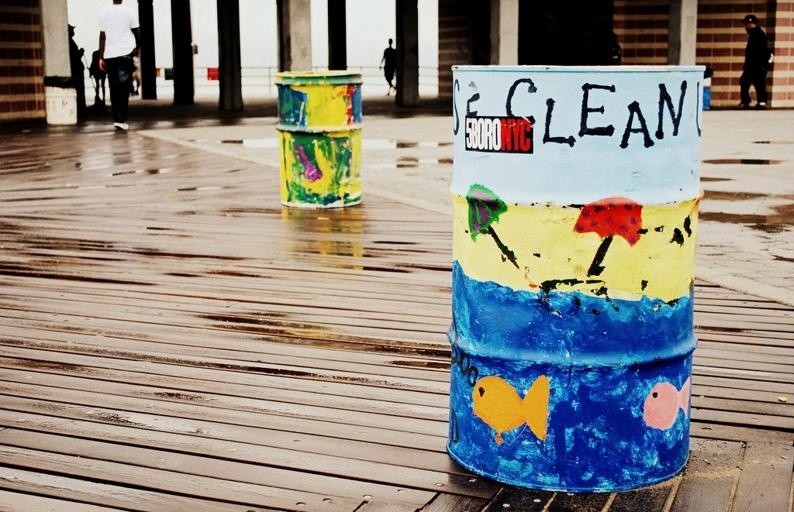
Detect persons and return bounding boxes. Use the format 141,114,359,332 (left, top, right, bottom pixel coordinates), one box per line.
87,49,107,103
378,38,396,96
129,49,140,97
734,12,774,110
67,24,89,129
96,0,143,135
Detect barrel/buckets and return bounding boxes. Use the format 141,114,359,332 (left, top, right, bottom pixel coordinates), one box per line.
273,69,363,210
445,62,705,497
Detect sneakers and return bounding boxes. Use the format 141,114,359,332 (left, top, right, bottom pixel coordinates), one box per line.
113,122,129,130
